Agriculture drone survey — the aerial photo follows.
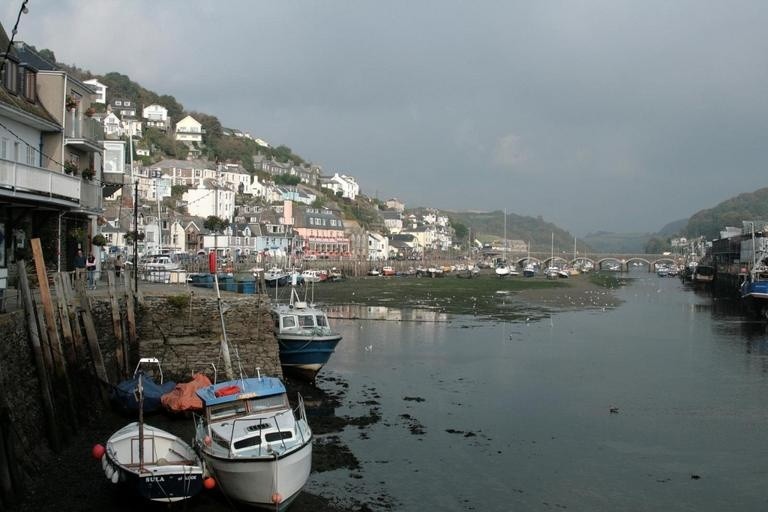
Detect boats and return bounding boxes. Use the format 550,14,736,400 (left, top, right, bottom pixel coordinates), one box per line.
143,255,178,269
494,263,569,279
262,265,344,386
738,244,768,317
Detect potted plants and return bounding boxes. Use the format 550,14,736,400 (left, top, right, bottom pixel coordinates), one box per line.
82,167,93,181
93,233,107,247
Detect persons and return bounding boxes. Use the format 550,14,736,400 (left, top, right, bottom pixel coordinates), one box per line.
86,250,97,290
74,249,86,280
114,256,121,277
188,246,196,258
225,248,269,266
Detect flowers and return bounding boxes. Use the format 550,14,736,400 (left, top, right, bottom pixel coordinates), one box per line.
65,159,77,170
65,94,78,111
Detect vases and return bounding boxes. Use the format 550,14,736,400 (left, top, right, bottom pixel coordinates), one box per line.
87,107,96,112
68,171,74,176
